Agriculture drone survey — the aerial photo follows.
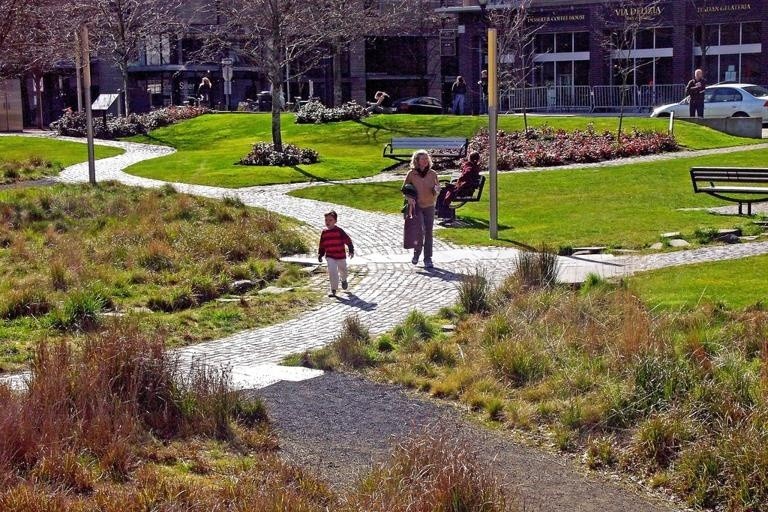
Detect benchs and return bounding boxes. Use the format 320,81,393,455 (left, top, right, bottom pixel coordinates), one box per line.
689,166,768,217
430,171,486,219
383,137,470,170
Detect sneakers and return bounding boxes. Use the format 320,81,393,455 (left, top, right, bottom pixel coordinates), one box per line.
328,289,336,297
340,278,348,290
424,257,434,269
412,254,419,264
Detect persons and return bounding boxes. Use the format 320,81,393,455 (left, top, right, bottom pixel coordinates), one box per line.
364,89,393,114
684,68,707,118
399,148,440,269
434,151,481,226
476,69,488,115
195,76,213,108
449,75,467,115
638,81,654,113
317,210,354,298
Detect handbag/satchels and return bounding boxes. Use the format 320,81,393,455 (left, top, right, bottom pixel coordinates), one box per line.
403,216,418,249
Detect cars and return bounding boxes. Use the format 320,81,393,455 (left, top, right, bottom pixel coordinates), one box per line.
649,81,768,129
390,96,452,114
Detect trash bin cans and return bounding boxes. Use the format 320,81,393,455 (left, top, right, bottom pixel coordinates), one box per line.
257,90,272,111
47,110,65,130
